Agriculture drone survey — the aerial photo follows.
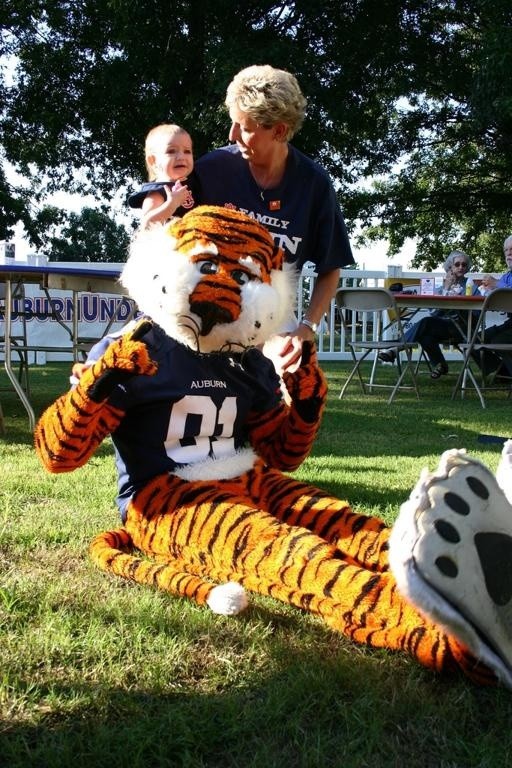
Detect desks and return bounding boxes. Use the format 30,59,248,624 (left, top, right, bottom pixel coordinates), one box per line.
0,265,137,433
370,293,488,399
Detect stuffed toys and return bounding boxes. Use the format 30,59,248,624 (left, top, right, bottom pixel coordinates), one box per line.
34,206,511,690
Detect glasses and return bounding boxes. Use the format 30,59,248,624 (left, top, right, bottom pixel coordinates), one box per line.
450,261,468,269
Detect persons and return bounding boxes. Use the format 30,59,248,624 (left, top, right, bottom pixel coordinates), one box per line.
468,235,512,386
88,63,356,521
378,251,480,379
142,123,195,224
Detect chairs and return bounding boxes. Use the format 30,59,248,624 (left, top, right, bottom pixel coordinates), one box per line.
0,282,31,402
451,287,512,409
334,287,421,406
77,296,136,345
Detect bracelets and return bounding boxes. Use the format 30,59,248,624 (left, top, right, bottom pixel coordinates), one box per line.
301,319,320,335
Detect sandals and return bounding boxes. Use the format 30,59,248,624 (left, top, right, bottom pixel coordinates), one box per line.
430,362,449,380
378,347,397,364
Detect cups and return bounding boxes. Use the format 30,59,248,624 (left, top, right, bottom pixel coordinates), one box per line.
27,254,46,266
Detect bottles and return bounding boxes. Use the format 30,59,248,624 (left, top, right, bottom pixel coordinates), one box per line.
465,276,472,296
